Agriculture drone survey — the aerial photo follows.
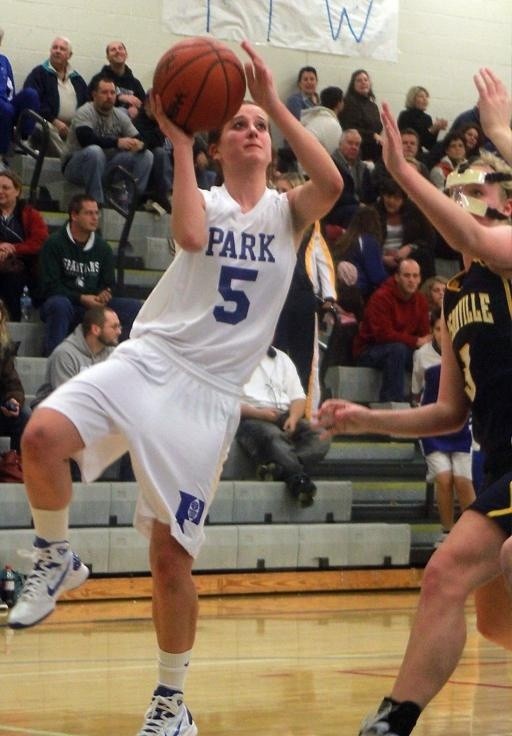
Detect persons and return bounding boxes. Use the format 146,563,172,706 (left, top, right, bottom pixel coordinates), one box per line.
317,99,512,736
1,36,511,548
3,41,344,736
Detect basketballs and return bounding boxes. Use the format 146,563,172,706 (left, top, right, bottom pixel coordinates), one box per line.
154,34,246,134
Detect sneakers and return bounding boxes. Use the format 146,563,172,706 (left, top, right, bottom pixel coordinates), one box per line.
7,542,89,627
136,687,197,736
0,449,23,484
296,477,316,509
258,462,276,482
